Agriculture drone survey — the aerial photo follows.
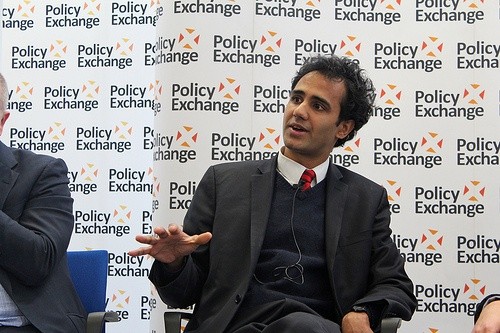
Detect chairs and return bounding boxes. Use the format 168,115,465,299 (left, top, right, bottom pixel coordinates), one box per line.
68,250,119,333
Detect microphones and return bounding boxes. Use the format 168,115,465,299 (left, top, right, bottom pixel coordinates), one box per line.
293,180,306,190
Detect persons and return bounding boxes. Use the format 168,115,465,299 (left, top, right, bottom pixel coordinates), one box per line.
471,295,500,333
128,54,419,333
0,73,88,333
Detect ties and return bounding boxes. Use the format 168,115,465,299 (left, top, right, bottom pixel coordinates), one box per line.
301,170,316,192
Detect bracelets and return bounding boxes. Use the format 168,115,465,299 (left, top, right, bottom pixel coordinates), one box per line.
351,306,368,313
